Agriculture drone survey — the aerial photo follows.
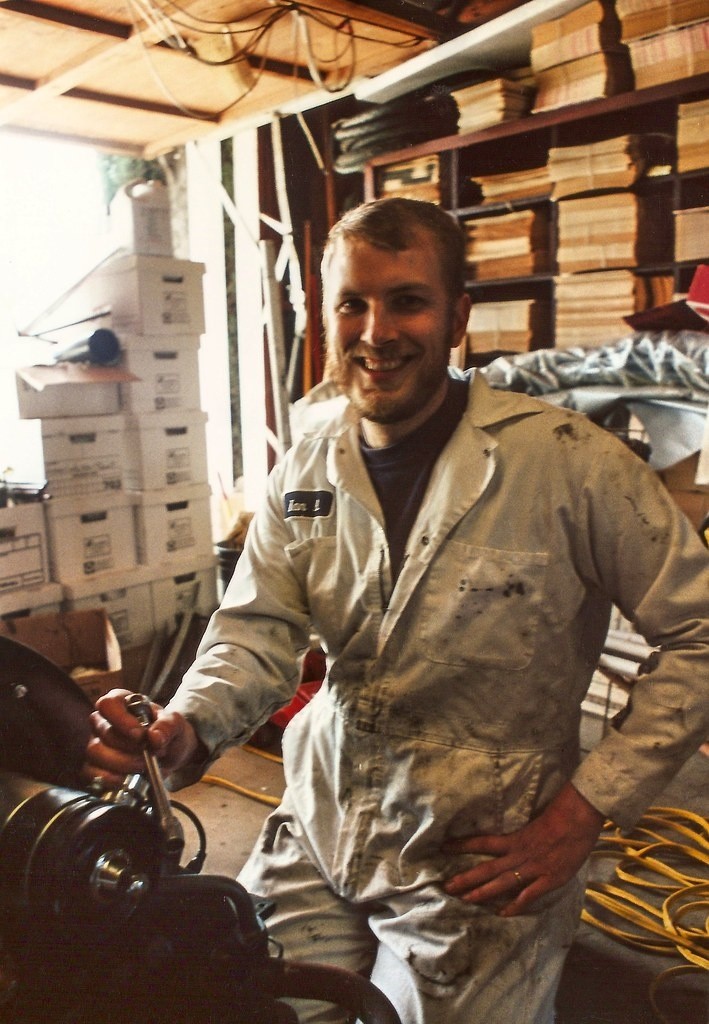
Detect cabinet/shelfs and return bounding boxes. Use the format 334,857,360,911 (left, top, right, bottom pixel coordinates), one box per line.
362,73,709,369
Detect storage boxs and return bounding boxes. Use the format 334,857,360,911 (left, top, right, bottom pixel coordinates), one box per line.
0,174,221,702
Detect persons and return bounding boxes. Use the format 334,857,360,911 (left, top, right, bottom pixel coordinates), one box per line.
81,197,709,1024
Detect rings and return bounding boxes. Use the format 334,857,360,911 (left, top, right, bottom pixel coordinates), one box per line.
512,868,523,885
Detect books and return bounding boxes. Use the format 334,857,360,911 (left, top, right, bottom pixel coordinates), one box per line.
380,1,709,719
